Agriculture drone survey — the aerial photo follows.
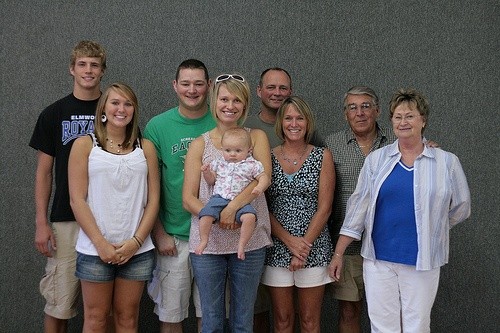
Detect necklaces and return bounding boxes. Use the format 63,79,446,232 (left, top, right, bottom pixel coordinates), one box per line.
358,145,367,147
280,144,308,165
106,134,123,153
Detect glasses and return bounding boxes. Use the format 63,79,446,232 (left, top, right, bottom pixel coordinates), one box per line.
346,103,377,111
393,112,422,120
215,74,245,88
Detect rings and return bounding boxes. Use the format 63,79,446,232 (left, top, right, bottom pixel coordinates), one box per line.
107,261,111,264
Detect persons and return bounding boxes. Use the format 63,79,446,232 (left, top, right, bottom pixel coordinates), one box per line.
194,127,270,261
269,95,337,333
182,74,273,333
326,88,471,333
29,39,142,333
67,82,159,333
320,85,439,333
242,67,326,333
143,58,246,333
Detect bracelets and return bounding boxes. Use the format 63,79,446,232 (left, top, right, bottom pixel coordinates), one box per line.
132,234,145,249
333,253,342,256
